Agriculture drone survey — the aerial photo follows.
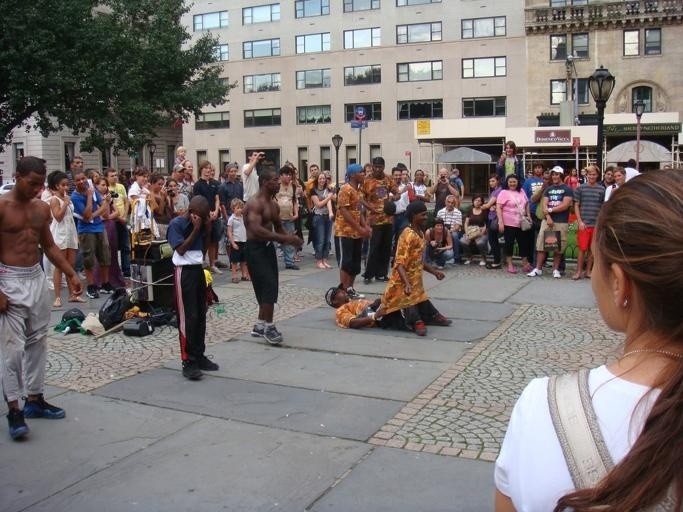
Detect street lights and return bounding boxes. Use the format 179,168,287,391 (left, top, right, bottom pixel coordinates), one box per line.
147,141,157,174
587,62,616,185
332,133,342,192
634,98,645,172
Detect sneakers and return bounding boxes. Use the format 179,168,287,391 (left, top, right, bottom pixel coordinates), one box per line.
181,360,203,380
285,263,300,270
374,275,390,283
5,406,29,440
552,269,562,279
323,262,333,269
251,323,263,337
486,263,503,269
209,265,224,275
261,324,284,345
122,271,131,280
526,267,545,277
231,277,239,284
196,354,220,372
240,275,252,281
201,261,210,270
345,286,366,300
505,265,517,273
66,295,87,304
293,254,301,262
22,395,66,421
296,251,305,257
363,277,372,285
464,259,473,265
277,251,284,257
435,262,445,270
413,319,428,336
51,296,62,307
479,261,487,266
519,264,534,273
425,312,453,327
99,282,116,295
215,260,228,269
85,284,100,299
315,262,327,269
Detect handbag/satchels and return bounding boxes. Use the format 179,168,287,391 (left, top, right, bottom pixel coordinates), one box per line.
519,214,534,232
543,229,562,250
304,210,315,232
466,224,482,241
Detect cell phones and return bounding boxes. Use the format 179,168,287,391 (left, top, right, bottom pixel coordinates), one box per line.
612,185,616,188
502,151,506,158
440,176,446,182
258,154,265,159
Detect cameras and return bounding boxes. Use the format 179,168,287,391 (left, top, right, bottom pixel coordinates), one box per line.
165,188,175,197
108,191,118,198
445,222,451,229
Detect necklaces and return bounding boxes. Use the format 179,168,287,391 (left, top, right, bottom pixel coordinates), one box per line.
623,348,682,362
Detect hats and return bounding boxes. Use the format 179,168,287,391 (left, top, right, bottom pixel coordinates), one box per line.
324,282,344,309
383,198,396,215
549,165,566,175
405,200,427,216
171,163,187,173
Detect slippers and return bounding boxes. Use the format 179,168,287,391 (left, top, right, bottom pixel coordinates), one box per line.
572,274,583,280
585,276,592,279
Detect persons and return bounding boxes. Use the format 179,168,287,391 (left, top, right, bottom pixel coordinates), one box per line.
0,156,84,439
37,142,640,381
492,168,682,512
